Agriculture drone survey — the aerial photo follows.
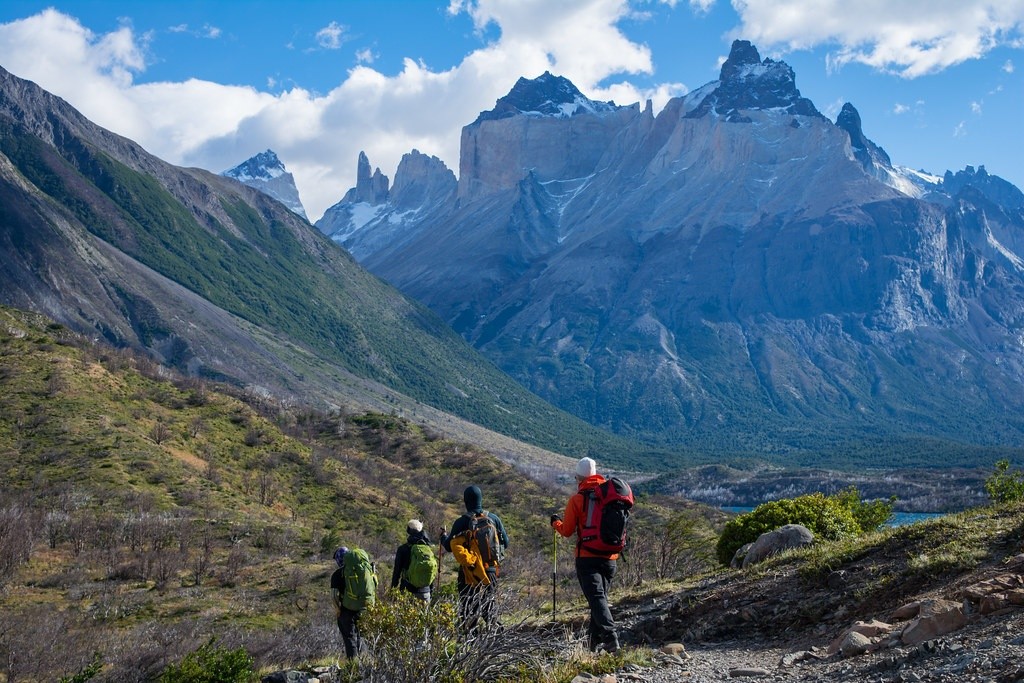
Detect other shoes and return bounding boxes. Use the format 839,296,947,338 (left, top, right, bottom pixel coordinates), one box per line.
598,639,620,652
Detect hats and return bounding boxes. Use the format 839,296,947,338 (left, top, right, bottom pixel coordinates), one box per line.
407,519,423,534
333,547,348,567
576,457,597,477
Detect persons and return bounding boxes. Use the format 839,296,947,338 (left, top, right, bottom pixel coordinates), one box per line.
439,485,509,637
548,457,622,653
330,547,378,663
391,519,438,651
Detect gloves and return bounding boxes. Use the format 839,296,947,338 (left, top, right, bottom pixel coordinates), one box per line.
550,515,562,526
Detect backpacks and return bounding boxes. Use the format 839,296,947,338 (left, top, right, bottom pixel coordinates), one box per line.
578,475,634,557
343,548,379,612
451,510,500,587
403,543,438,588
